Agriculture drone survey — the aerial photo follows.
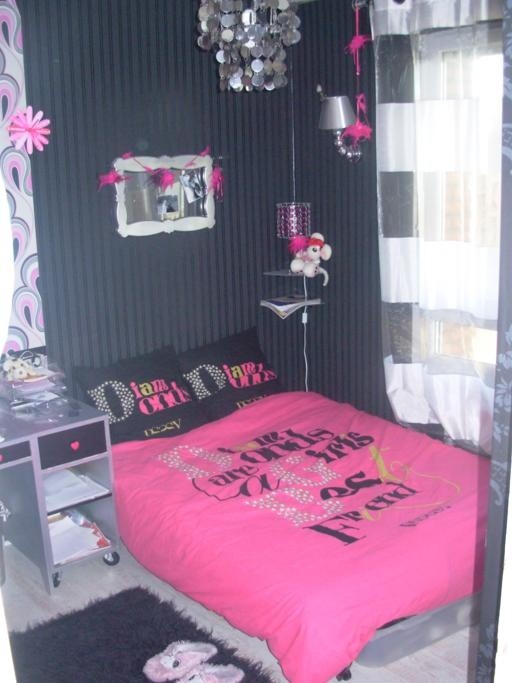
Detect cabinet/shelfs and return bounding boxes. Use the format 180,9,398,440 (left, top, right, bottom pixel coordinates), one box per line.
261,271,321,310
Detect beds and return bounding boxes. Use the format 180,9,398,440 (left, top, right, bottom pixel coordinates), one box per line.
105,385,491,683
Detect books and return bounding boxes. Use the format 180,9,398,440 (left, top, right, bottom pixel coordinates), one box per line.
262,303,305,319
261,294,320,310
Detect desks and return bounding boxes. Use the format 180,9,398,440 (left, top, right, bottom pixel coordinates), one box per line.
0,397,118,597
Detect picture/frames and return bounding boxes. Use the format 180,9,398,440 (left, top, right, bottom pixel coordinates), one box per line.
113,153,218,237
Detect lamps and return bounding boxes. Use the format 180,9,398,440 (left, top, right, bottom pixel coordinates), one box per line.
318,96,363,165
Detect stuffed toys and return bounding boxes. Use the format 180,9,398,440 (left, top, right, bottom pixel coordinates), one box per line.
290,231,332,286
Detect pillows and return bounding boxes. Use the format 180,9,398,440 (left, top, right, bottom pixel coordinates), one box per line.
68,325,287,447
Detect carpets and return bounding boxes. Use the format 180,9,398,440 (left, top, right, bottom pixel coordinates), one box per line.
7,582,271,683
